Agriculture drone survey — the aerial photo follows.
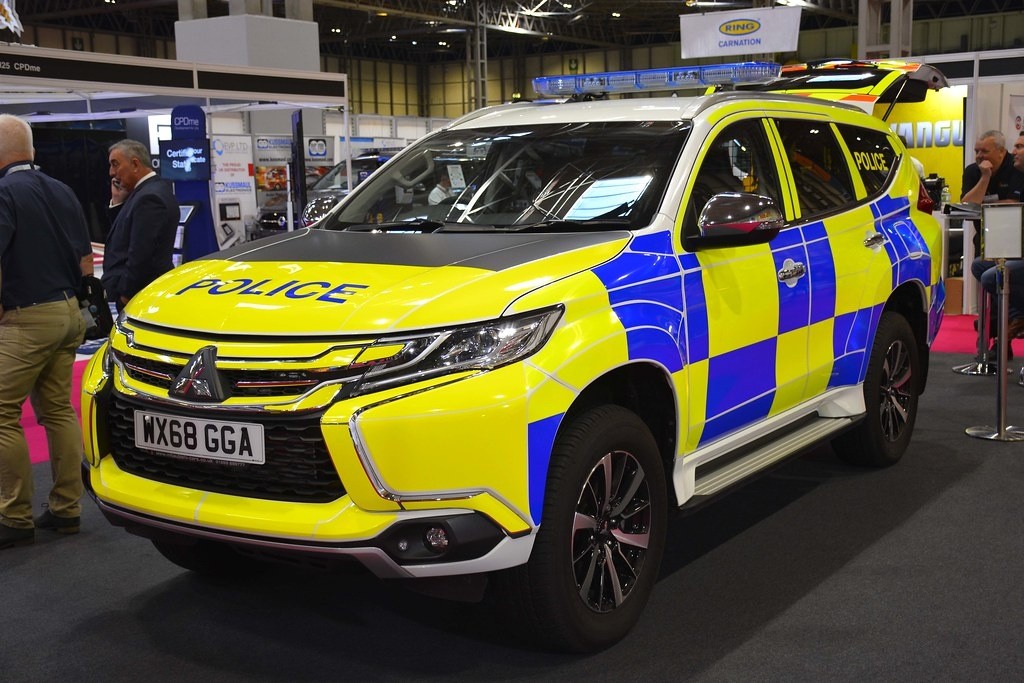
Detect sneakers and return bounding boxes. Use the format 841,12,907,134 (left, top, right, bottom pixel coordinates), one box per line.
0,522,35,549
34,503,81,535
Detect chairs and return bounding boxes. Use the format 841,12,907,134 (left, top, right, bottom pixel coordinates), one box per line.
684,132,838,237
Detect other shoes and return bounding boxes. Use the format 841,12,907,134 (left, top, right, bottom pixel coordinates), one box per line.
974,317,999,339
974,341,1013,362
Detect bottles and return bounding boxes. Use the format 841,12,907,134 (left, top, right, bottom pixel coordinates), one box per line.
941,183,949,214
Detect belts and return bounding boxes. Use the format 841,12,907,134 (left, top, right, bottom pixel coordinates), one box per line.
3,290,76,312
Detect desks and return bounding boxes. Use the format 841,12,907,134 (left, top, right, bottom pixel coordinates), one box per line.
942,209,1014,377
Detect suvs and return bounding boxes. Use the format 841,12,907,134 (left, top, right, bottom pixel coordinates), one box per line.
83,56,952,656
251,148,410,240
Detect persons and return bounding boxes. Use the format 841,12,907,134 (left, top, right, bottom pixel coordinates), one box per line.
960,130,1024,361
102,140,181,312
428,171,456,206
0,114,94,547
897,135,925,178
